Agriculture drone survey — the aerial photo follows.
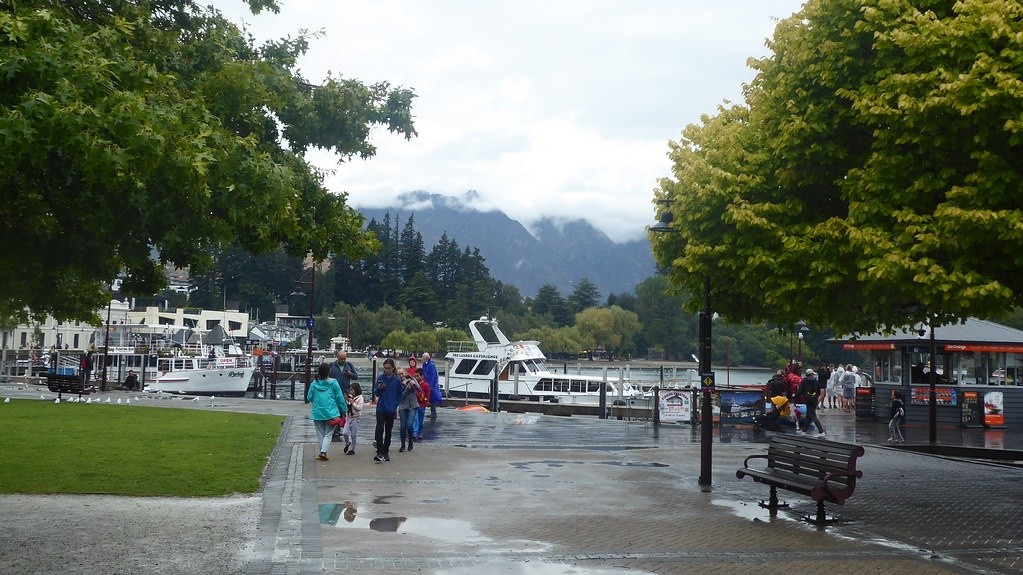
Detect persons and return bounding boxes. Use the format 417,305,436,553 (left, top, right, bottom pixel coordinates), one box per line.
373,360,403,462
328,350,358,442
341,383,364,456
916,363,941,378
85,352,93,384
122,370,139,392
767,360,822,433
396,352,442,451
307,363,348,461
887,390,905,443
818,363,873,413
208,349,218,370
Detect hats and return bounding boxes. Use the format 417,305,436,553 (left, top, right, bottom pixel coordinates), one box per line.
806,369,816,375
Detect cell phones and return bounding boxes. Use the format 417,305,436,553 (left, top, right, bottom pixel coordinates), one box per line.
378,380,386,387
406,380,410,385
345,369,348,372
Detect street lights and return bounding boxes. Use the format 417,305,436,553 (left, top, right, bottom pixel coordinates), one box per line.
916,320,937,444
289,262,315,405
798,326,810,360
648,198,714,483
790,319,806,363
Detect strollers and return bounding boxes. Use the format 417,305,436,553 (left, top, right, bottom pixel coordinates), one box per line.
752,395,795,434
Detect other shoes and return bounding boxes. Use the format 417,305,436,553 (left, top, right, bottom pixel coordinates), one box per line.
374,454,383,461
829,405,832,408
343,442,351,453
316,456,321,460
418,433,423,439
413,434,417,440
333,437,342,442
384,454,389,460
319,453,329,460
817,406,820,409
346,450,355,454
427,414,437,418
895,437,904,442
821,405,827,408
408,444,413,451
399,447,405,452
834,405,837,408
887,438,894,442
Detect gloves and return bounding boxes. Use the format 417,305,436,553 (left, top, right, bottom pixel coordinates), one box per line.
378,383,386,390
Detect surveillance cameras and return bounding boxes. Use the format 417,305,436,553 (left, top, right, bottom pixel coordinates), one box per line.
917,329,926,336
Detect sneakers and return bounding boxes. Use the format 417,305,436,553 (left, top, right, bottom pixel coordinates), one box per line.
796,429,806,437
814,432,825,437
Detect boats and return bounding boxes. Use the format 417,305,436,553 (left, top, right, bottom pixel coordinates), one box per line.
436,308,699,406
90,287,256,397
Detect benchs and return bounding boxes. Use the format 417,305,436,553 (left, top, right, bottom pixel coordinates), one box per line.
736,432,865,522
47,374,96,403
